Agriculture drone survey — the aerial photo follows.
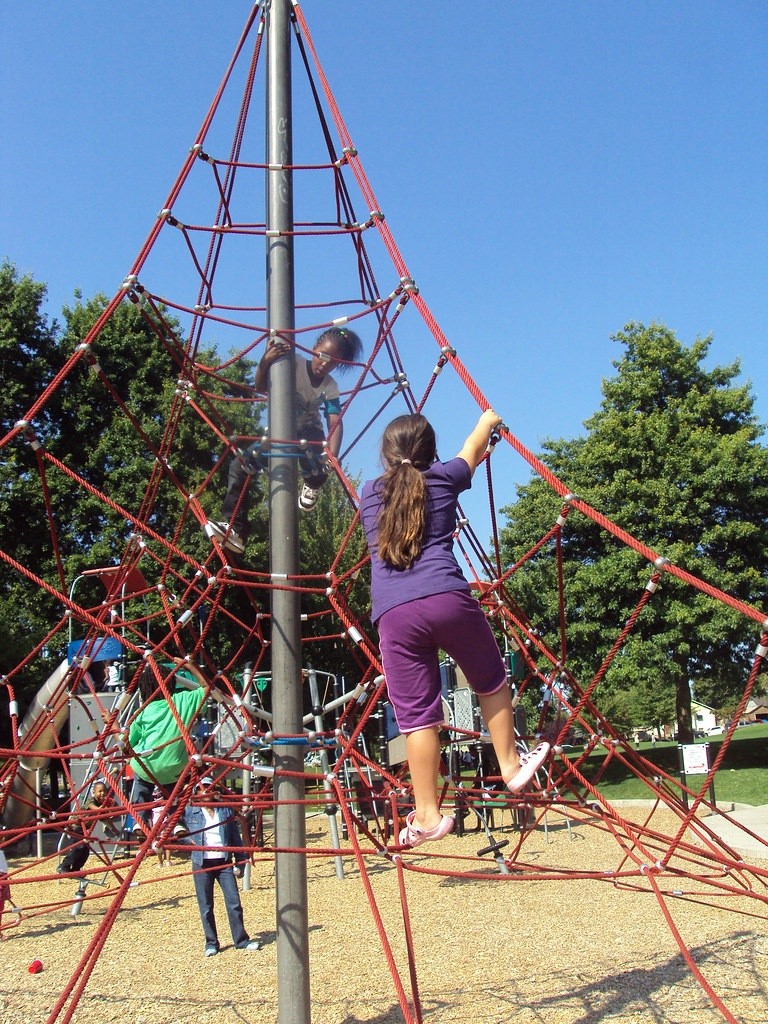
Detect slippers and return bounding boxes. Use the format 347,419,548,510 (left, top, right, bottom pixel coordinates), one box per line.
399,810,454,849
506,741,550,794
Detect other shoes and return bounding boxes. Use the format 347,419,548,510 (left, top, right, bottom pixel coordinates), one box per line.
206,947,217,957
133,823,147,843
173,822,187,836
236,942,259,950
56,866,86,878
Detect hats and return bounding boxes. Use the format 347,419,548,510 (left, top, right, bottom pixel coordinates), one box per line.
193,777,214,792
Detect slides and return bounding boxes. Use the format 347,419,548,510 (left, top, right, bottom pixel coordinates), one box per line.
0,657,96,848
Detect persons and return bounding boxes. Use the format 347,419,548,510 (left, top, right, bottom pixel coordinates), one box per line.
102,657,210,842
168,777,259,957
56,781,107,879
103,659,122,692
652,733,656,747
207,326,364,553
635,732,640,750
361,409,549,847
0,824,11,942
441,749,474,770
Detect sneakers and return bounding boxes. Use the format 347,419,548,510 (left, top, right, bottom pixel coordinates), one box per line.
206,521,245,554
298,483,318,512
